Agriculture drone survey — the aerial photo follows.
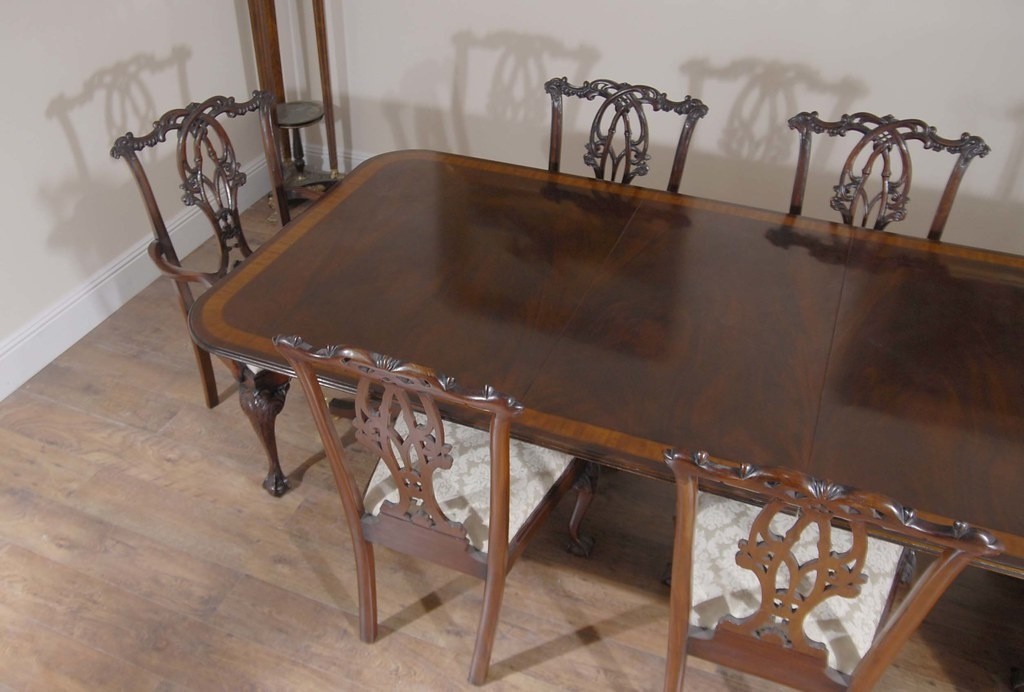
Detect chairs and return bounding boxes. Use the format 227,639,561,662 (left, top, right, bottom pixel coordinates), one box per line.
786,111,993,241
270,331,598,685
545,76,710,191
665,448,1005,692
108,90,342,497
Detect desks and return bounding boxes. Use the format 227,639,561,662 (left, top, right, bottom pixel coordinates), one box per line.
188,147,1024,692
